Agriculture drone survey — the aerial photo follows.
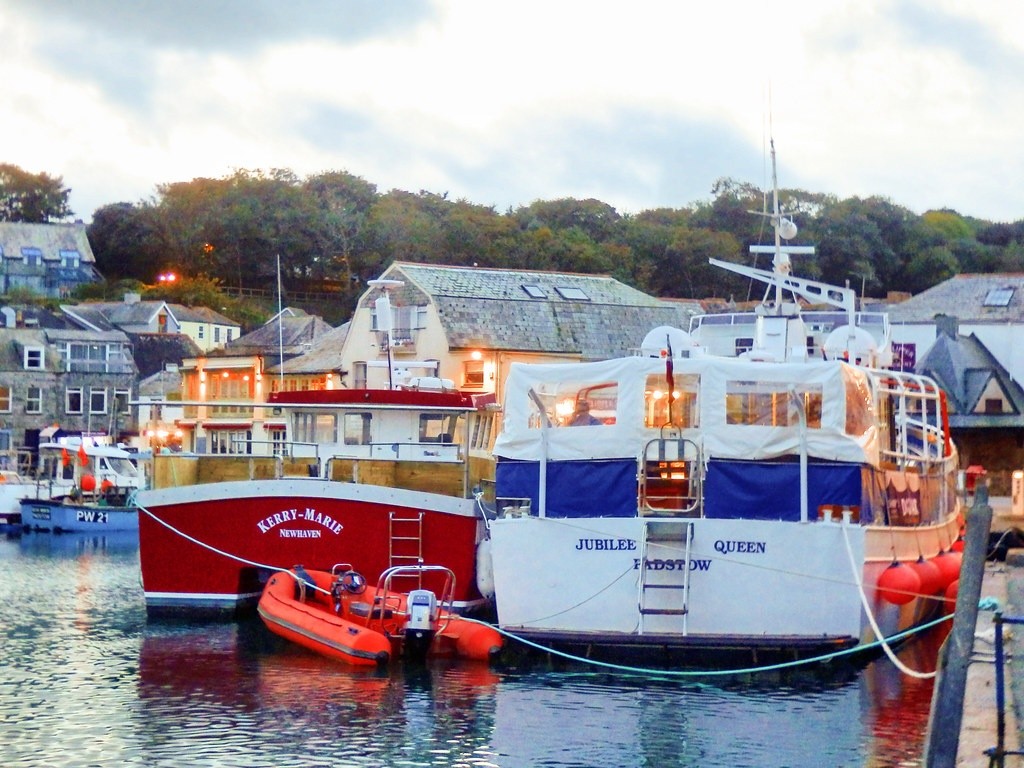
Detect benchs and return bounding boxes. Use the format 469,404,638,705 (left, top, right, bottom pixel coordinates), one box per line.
350,601,392,635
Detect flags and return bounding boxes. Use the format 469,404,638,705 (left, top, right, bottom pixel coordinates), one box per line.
666,335,676,404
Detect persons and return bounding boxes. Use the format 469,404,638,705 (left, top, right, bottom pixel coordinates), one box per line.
568,400,601,426
71,484,78,502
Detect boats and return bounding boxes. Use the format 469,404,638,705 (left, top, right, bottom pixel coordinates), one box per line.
257,564,502,667
131,385,499,619
19,442,140,530
488,140,966,652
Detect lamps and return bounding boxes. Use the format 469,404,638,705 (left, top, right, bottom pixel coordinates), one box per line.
273,406,281,415
